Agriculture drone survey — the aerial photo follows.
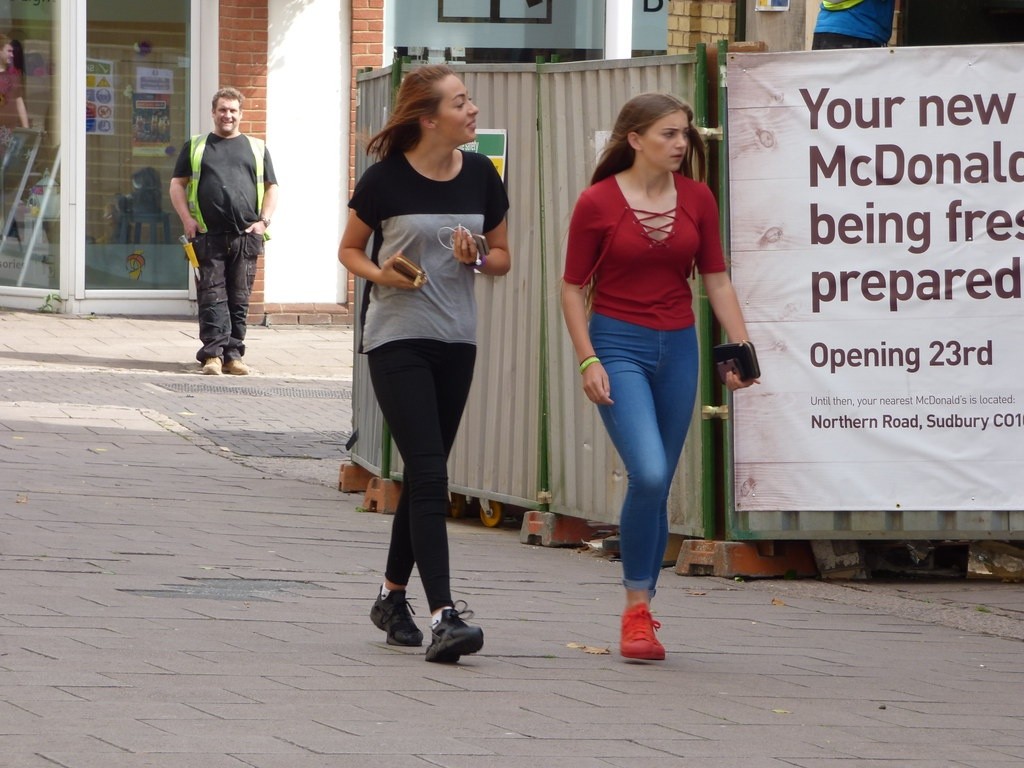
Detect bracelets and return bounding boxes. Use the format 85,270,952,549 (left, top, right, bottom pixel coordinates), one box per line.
580,354,601,374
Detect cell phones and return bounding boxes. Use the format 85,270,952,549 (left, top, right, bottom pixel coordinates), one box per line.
717,358,746,385
472,234,490,256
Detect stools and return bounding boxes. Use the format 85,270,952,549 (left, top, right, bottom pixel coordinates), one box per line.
118,210,172,245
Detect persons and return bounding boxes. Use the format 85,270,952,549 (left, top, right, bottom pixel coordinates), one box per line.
561,89,762,662
811,1,897,49
340,62,513,661
0,35,30,166
169,87,279,376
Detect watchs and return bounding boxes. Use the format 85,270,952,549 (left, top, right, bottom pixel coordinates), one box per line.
260,218,270,227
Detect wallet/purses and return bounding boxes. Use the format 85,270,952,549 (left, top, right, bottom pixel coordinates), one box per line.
712,342,761,383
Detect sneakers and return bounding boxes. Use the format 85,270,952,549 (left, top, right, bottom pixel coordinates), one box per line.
370,584,423,646
620,602,665,664
222,359,252,375
203,356,222,375
425,600,484,664
